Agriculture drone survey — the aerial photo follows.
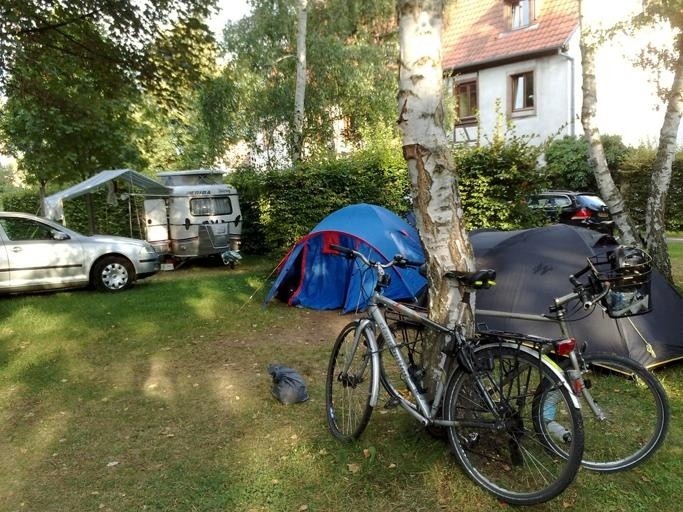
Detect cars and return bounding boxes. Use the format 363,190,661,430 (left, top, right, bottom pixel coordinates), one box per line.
527,188,612,237
0,209,158,302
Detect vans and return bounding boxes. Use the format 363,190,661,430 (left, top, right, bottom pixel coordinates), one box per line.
138,170,246,267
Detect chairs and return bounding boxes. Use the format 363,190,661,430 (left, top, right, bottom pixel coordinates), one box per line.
544,198,557,208
528,197,540,206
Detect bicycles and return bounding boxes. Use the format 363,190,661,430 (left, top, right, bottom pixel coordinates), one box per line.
372,239,669,475
320,241,583,505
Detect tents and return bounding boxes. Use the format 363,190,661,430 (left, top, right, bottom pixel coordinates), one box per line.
469,224,683,378
265,203,428,316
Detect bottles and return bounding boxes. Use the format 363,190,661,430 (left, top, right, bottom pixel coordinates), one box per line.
543,380,563,422
547,421,572,443
